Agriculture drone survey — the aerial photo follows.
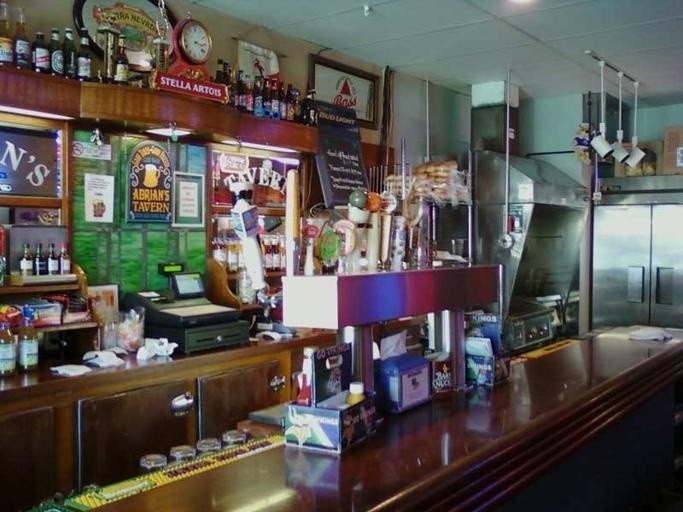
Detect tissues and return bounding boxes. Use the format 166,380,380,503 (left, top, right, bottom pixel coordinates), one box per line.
371,328,431,415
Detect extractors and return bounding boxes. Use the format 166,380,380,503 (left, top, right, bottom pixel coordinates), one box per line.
473,151,588,207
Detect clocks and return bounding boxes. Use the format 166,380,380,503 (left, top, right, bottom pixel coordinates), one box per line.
176,19,212,65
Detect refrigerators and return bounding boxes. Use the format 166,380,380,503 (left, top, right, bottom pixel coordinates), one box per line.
590,204,681,333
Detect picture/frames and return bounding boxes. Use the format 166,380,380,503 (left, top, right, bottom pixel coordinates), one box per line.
72,0,179,70
307,53,380,131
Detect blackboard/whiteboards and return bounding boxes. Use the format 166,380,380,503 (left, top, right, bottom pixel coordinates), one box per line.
304,97,371,210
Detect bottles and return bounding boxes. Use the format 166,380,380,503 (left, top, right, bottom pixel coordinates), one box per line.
138,429,246,477
214,58,318,127
295,345,320,407
18,315,38,370
1,322,15,376
210,233,285,276
345,382,365,405
0,1,91,81
110,33,129,86
18,241,71,275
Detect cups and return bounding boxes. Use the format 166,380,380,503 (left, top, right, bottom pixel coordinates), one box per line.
114,305,146,352
